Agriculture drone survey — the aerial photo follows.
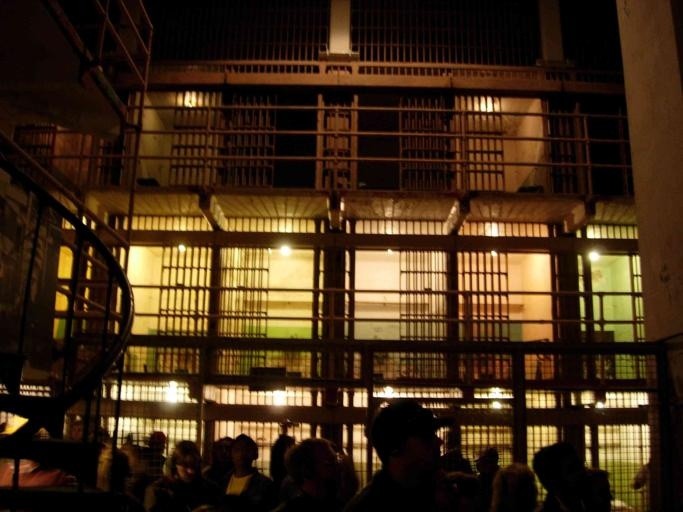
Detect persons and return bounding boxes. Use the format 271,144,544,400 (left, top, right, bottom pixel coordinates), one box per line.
1,419,652,512
339,398,469,510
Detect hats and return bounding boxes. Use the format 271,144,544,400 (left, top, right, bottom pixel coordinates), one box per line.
225,433,258,461
364,399,454,446
